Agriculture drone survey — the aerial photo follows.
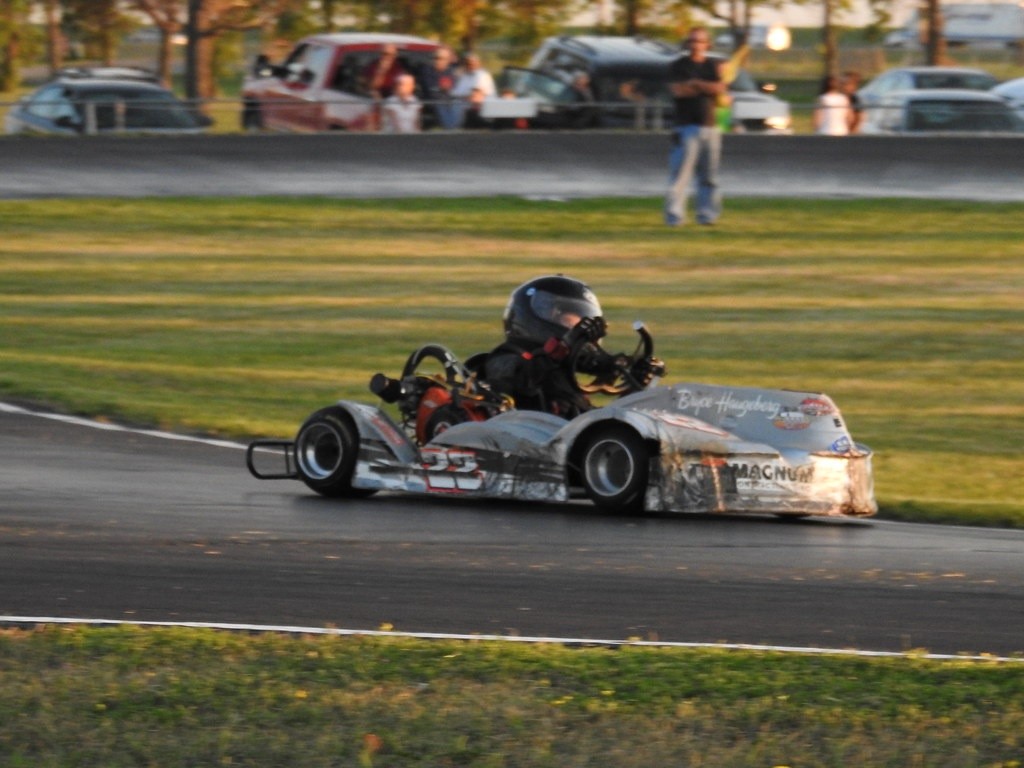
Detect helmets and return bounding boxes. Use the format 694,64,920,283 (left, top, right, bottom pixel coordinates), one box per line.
503,274,603,349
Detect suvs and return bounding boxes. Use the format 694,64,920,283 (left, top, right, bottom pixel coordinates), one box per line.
6,64,222,134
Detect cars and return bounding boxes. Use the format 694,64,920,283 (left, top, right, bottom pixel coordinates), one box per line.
853,67,1004,130
986,74,1024,130
514,36,682,131
684,50,796,136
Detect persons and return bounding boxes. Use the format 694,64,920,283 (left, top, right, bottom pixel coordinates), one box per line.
813,72,866,136
663,27,728,228
480,273,668,422
367,42,527,131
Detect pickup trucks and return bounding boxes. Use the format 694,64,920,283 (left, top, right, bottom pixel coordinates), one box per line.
237,33,459,135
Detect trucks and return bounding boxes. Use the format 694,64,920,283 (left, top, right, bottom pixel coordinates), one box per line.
883,3,1023,60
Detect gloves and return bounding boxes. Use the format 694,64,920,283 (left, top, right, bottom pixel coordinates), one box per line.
561,316,606,351
626,353,666,386
